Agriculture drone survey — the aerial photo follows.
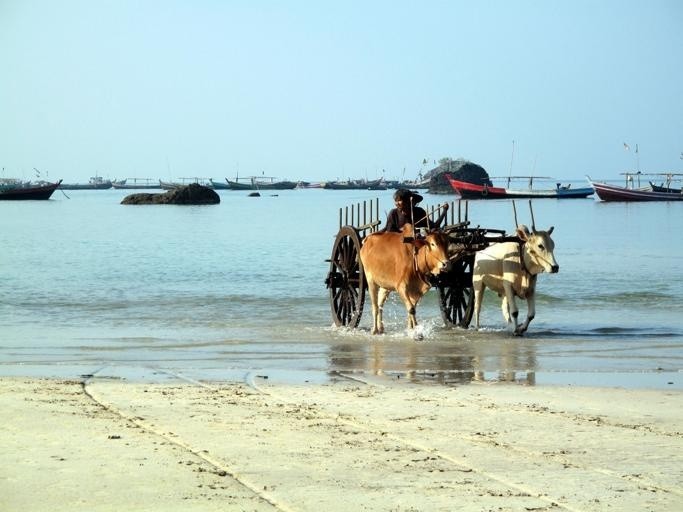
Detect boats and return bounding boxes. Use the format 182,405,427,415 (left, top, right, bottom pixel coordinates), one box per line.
441,170,594,200
0,168,63,201
45,176,115,189
159,169,433,200
111,177,162,190
590,180,683,202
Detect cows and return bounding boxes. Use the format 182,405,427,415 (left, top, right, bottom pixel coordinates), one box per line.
360,223,452,340
472,225,559,336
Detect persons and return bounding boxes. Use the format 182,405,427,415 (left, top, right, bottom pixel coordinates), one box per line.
386,188,449,233
